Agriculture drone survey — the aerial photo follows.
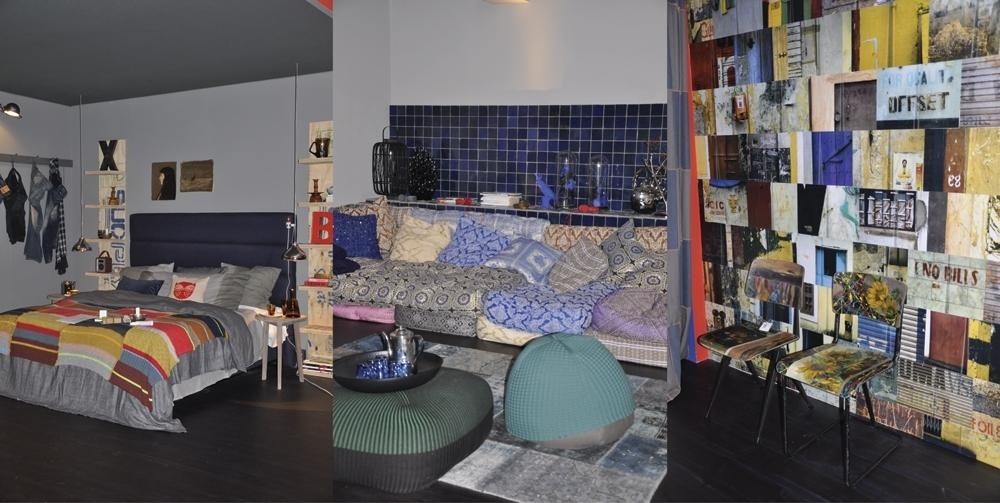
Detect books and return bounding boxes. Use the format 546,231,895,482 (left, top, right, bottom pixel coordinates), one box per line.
56,313,98,325
479,191,523,206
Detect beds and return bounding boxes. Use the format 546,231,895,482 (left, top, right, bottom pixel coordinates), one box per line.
0,210,298,424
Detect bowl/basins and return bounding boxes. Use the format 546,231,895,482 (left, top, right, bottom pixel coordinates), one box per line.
97,229,112,239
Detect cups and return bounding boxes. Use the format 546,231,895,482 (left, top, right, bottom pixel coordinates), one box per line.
70,289,80,295
354,356,416,380
282,299,289,315
268,302,276,314
308,137,330,157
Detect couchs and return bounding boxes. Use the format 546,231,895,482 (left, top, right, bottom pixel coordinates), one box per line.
332,201,668,371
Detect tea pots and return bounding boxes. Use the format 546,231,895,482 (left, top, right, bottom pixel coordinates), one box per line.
380,324,424,370
629,165,665,213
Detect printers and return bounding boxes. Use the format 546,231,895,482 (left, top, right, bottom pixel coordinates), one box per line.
96,257,112,273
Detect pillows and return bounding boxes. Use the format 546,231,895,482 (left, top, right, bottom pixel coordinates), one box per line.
116,259,283,310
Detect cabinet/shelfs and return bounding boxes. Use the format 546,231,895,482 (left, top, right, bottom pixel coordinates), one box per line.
297,117,333,379
81,138,126,290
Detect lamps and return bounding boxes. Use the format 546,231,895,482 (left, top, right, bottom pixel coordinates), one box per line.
283,214,307,317
0,101,23,120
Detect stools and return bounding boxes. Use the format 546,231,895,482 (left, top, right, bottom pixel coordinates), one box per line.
504,332,637,451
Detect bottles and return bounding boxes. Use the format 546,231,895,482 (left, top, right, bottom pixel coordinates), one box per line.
286,289,301,318
133,307,142,321
63,280,71,294
117,188,124,202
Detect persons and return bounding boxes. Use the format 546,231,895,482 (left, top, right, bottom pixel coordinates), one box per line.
895,159,914,186
152,166,176,200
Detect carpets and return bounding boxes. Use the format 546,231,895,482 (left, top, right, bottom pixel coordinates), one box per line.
333,329,667,501
